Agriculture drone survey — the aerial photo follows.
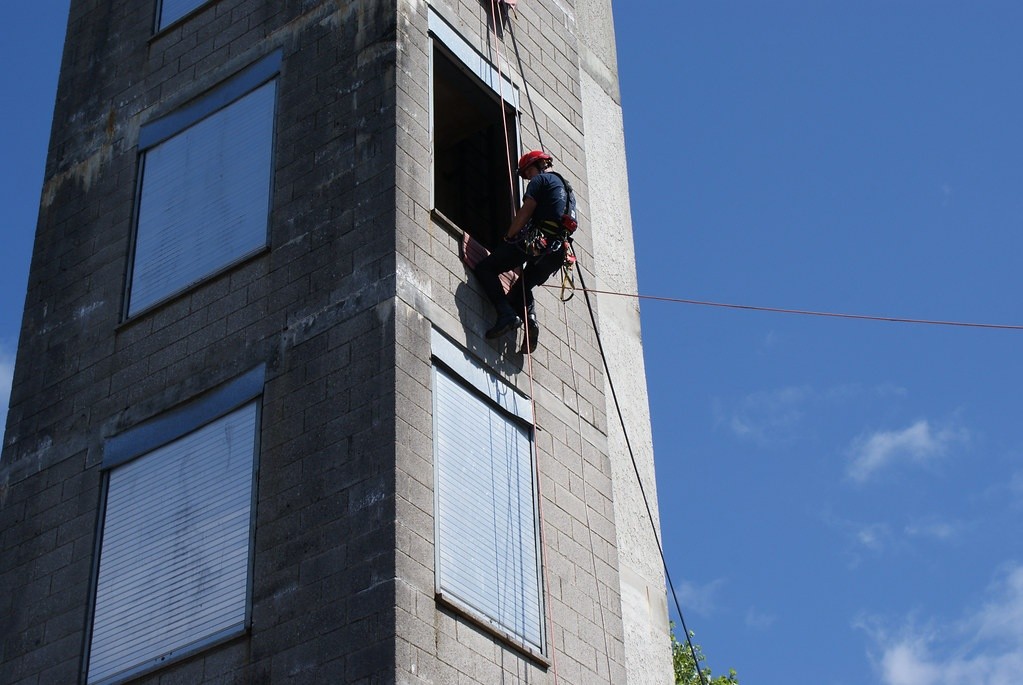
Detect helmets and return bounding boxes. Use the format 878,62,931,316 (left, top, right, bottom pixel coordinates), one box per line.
517,151,553,179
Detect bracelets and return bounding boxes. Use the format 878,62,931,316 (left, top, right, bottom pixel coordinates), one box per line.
504,232,512,241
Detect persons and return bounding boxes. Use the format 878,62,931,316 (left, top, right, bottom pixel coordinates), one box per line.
475,151,576,354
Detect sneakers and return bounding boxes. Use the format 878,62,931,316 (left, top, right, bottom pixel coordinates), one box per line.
521,320,539,354
486,315,522,338
